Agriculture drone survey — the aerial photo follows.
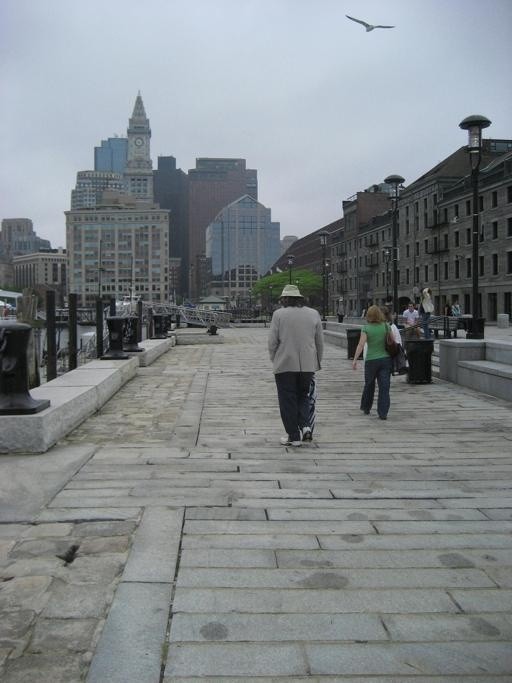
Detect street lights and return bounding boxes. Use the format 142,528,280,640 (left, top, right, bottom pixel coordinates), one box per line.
286,254,296,284
384,175,406,328
318,230,330,321
458,114,492,340
269,285,273,303
248,289,252,304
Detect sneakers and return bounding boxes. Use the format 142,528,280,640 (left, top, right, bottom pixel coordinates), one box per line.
281,427,312,447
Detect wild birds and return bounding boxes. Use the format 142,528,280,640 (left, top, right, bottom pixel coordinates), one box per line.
343,13,395,32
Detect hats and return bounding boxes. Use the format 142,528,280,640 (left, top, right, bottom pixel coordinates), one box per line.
280,285,304,297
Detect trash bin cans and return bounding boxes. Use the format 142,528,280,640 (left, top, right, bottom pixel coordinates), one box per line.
346,329,363,360
404,339,434,384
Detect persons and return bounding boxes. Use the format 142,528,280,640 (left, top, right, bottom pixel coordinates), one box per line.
443,298,460,321
403,286,434,337
352,304,402,421
266,284,324,449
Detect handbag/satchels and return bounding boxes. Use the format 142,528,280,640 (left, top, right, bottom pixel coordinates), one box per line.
385,333,408,376
